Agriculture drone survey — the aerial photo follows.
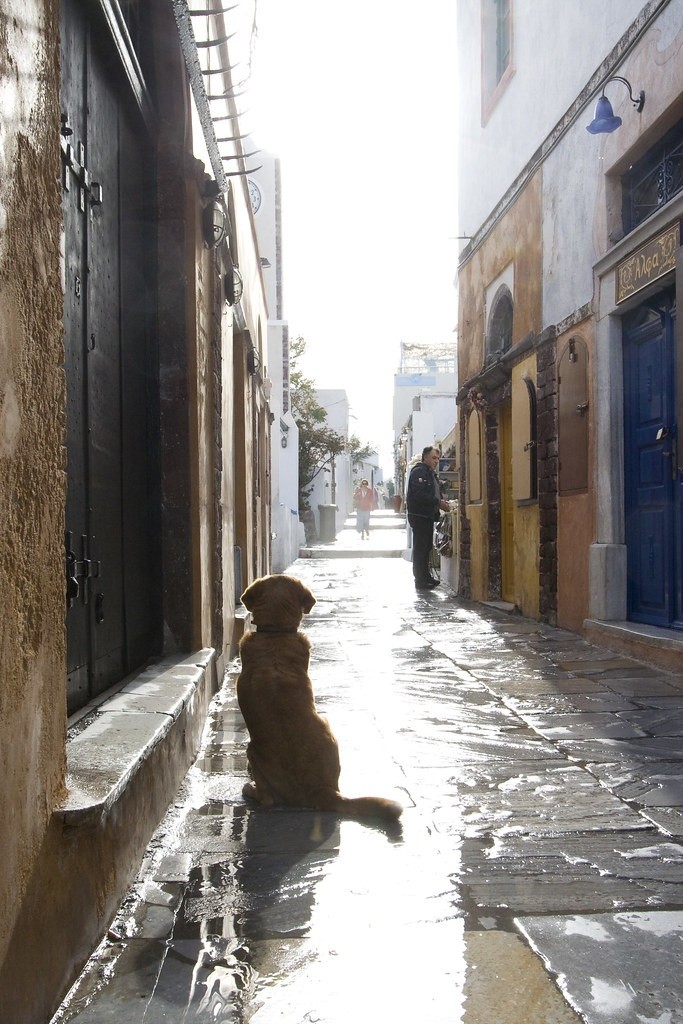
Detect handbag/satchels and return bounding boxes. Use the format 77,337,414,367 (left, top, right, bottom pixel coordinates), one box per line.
433,512,453,558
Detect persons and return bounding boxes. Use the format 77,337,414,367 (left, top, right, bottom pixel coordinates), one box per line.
354,481,372,541
407,446,451,590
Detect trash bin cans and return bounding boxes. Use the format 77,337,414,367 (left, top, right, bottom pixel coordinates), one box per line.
318,503,338,541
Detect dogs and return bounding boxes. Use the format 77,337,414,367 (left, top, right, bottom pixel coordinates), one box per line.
236,575,404,819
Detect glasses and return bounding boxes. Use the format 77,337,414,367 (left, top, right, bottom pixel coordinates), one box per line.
362,483,367,485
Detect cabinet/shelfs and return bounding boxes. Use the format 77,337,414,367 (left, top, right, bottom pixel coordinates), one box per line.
436,456,459,501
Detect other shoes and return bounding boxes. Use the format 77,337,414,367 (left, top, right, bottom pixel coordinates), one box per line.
415,580,435,590
426,576,440,587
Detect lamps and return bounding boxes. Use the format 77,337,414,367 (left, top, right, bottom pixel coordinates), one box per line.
586,75,645,135
201,198,262,376
